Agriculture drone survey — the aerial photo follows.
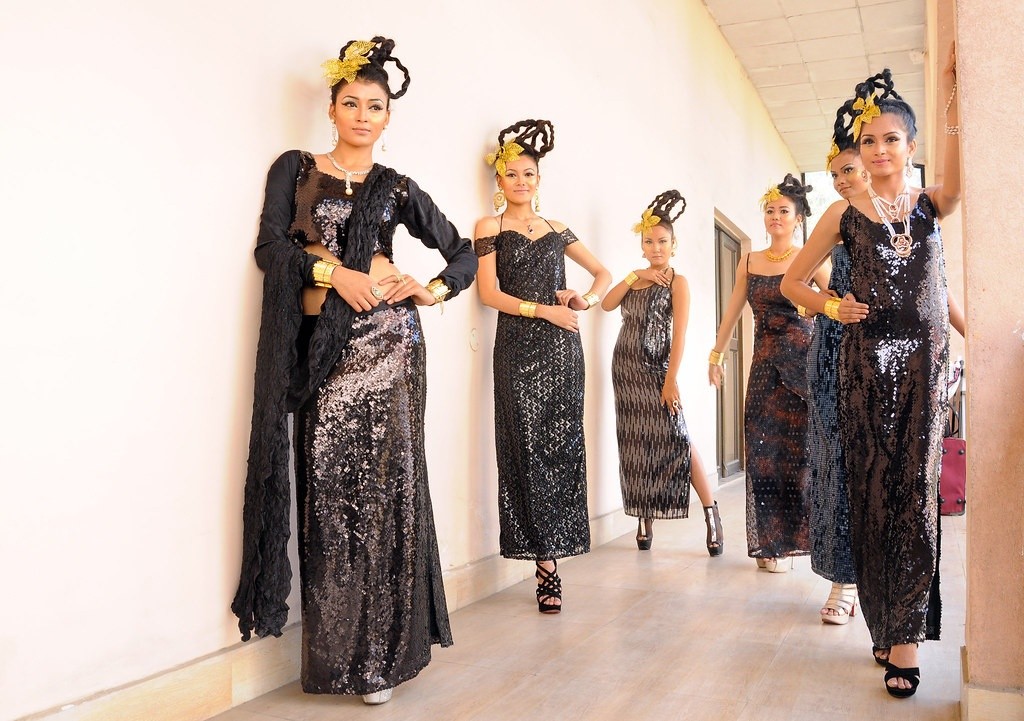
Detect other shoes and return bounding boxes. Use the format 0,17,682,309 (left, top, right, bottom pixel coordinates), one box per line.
363,687,392,705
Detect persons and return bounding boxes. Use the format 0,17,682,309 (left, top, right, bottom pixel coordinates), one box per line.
474,118,613,612
231,33,479,703
810,67,960,700
789,99,965,622
602,190,724,556
708,173,830,572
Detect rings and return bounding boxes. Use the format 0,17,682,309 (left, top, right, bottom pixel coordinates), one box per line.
673,400,679,407
370,286,384,300
391,274,403,283
401,280,407,286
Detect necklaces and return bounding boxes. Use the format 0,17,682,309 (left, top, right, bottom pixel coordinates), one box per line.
509,209,535,235
765,245,794,262
867,183,914,255
326,152,372,196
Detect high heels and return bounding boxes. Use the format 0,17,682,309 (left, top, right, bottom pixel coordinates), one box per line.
636,516,653,550
872,639,920,697
703,499,723,556
535,558,563,613
756,554,794,573
820,582,857,625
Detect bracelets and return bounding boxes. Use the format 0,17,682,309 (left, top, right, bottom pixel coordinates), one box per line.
625,271,639,286
425,279,451,315
708,350,724,367
943,84,959,136
824,298,843,322
581,291,601,311
312,259,339,288
520,300,538,318
797,305,806,317
